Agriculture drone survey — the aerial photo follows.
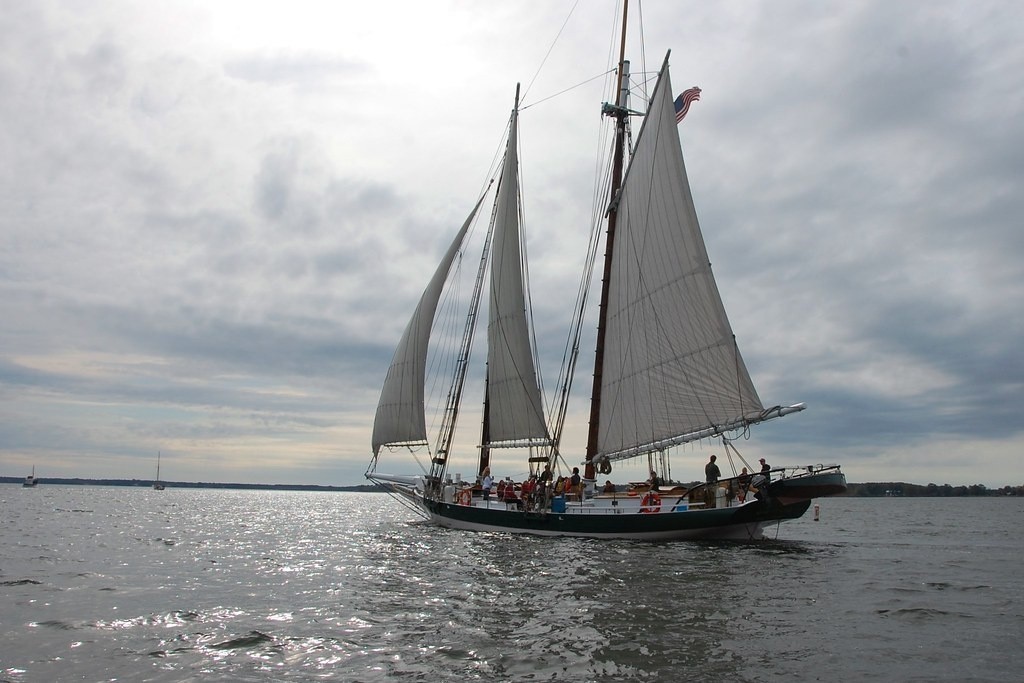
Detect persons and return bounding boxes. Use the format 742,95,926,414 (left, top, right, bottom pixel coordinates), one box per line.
758,458,771,480
648,470,659,492
481,465,580,510
704,455,750,500
603,480,614,492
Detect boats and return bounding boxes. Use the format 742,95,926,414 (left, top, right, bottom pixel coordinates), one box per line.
153,450,165,490
23,465,38,487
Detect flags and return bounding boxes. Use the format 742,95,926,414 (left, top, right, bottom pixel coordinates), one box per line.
674,86,702,124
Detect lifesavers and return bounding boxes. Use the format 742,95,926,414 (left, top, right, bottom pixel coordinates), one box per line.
459,489,472,506
641,493,661,513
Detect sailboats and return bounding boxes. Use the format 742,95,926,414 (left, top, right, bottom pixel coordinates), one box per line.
364,0,848,540
